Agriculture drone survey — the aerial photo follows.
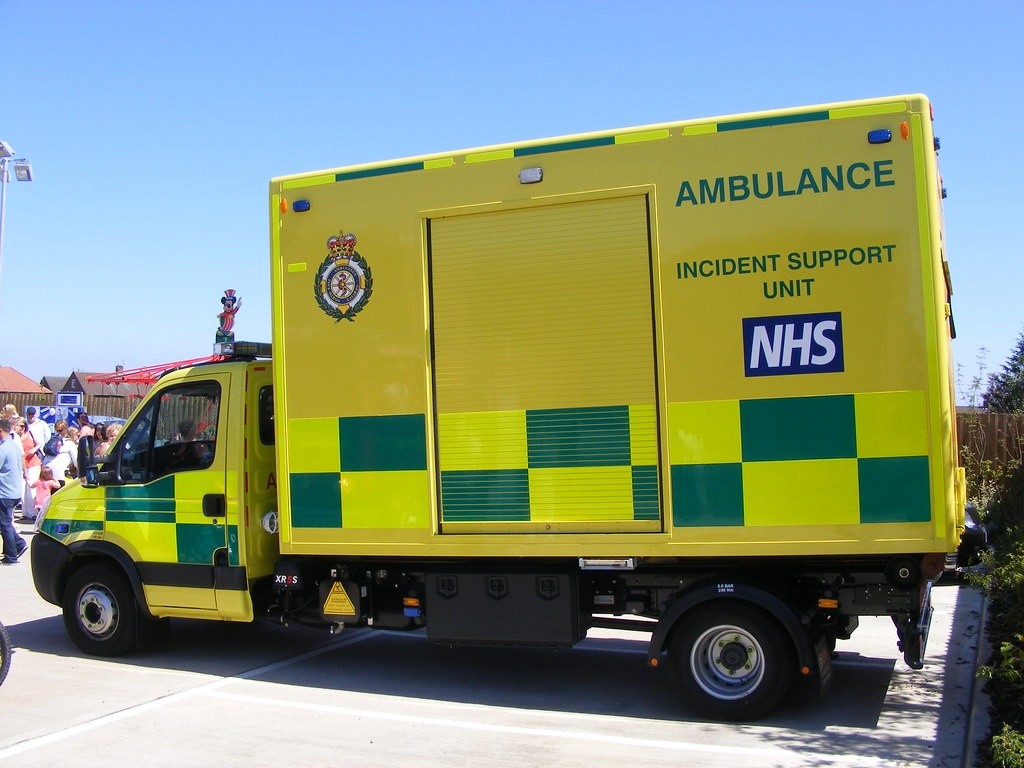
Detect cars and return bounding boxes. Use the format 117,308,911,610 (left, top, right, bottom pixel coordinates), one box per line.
13,416,150,507
566,504,989,615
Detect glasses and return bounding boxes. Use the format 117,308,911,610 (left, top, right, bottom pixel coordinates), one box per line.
55,429,62,433
27,413,35,416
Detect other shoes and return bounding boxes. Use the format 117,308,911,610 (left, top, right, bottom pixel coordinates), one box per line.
20,516,26,520
32,516,36,520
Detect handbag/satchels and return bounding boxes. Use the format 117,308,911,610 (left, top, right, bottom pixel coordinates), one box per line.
29,431,44,461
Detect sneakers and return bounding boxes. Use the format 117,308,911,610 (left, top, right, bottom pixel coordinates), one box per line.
16,544,29,558
0,557,19,564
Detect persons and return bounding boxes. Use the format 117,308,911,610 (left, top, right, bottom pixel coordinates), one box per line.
0,404,123,496
26,465,61,513
14,416,42,520
156,420,216,445
0,418,29,566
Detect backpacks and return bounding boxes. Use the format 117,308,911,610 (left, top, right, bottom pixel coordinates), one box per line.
44,433,64,456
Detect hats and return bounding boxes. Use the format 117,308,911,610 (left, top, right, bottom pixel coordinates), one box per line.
25,407,36,414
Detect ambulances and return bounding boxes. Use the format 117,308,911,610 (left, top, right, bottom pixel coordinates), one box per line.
31,91,964,724
